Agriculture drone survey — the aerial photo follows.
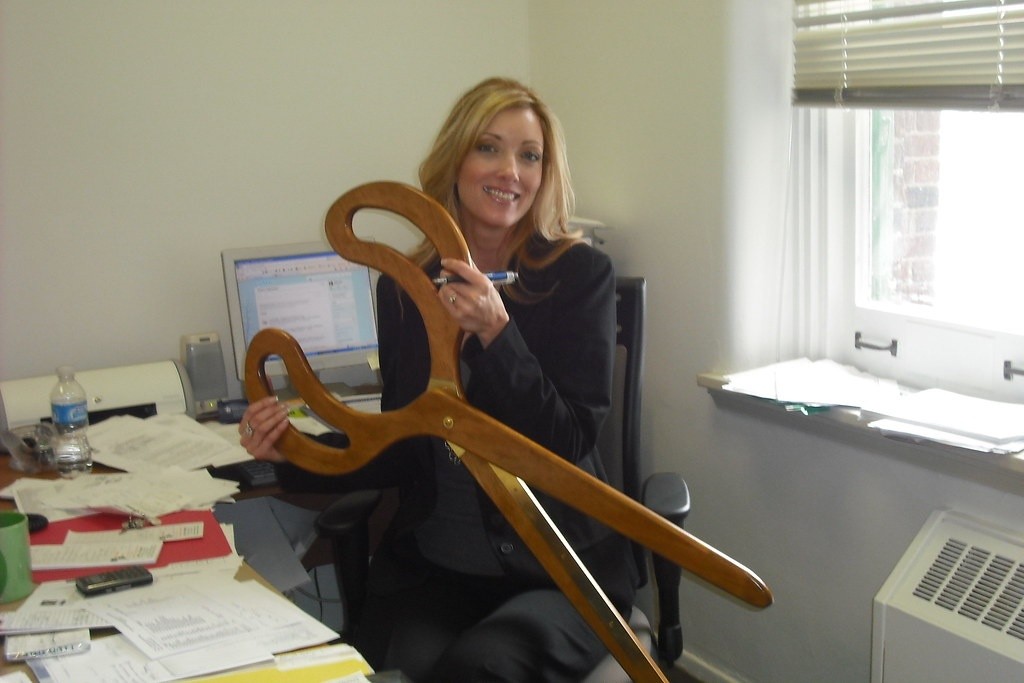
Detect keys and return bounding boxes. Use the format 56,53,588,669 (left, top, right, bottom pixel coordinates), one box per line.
119,510,162,535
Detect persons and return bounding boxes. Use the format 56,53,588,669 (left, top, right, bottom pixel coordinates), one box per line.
247,77,642,681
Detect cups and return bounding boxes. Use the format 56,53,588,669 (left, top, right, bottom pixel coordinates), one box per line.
0,511,34,604
6,424,55,473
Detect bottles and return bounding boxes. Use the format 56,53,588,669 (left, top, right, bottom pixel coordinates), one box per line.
51,365,93,478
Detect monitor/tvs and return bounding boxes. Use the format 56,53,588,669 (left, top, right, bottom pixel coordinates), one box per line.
221,240,379,409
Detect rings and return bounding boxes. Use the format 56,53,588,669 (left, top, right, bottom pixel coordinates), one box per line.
448,292,458,303
245,422,255,434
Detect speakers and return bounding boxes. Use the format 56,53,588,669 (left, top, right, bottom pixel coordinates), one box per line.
181,335,229,420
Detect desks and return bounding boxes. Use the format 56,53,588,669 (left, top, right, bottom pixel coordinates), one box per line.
0,381,383,683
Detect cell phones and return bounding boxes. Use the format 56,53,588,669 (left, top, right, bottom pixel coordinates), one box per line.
75,566,153,597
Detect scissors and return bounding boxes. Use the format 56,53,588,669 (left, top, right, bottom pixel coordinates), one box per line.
242,178,774,683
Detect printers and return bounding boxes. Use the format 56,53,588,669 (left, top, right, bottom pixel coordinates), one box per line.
0,360,186,452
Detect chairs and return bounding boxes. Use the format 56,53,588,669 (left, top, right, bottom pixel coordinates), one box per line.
317,274,694,683
